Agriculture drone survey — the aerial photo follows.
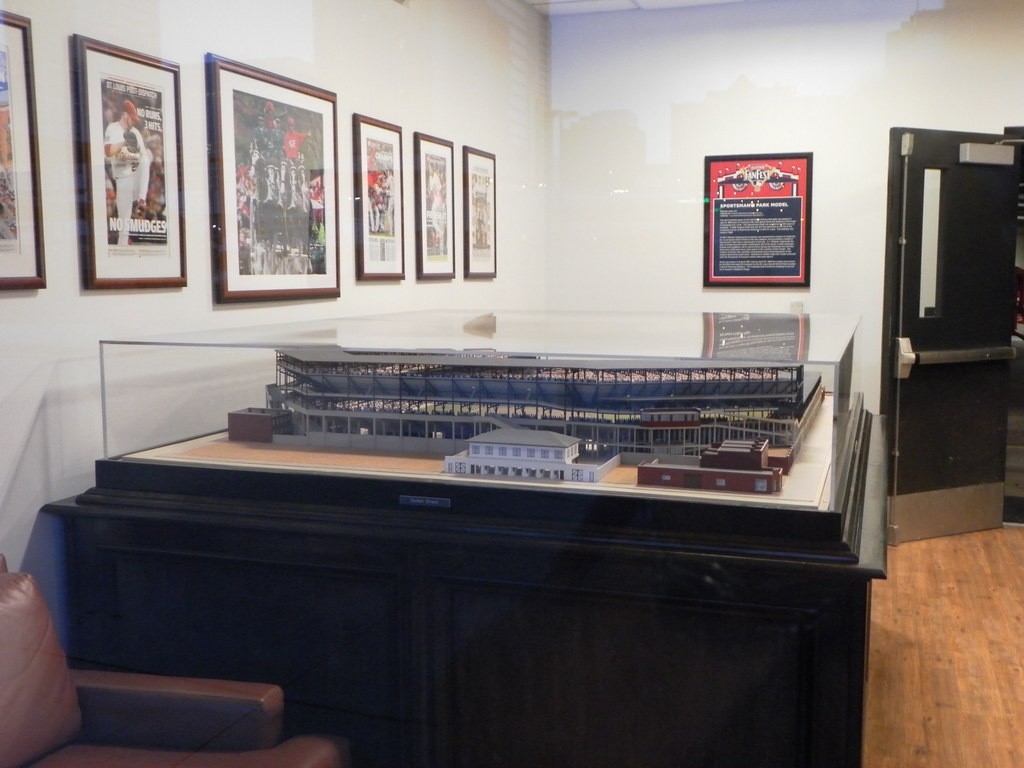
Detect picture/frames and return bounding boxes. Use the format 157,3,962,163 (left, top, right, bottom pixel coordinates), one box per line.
206,52,341,303
352,113,405,282
73,34,188,290
413,131,457,280
0,9,50,290
462,145,497,279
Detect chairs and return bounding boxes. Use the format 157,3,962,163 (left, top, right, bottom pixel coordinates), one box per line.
0,553,351,768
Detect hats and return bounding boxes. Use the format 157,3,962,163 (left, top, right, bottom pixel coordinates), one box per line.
287,116,294,125
256,112,265,121
122,100,140,122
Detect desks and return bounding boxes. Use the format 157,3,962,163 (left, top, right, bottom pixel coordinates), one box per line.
39,311,887,768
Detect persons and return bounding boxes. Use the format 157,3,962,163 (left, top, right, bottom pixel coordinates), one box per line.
106,99,150,245
237,102,490,276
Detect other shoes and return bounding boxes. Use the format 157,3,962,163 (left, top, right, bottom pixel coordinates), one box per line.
137,204,145,217
